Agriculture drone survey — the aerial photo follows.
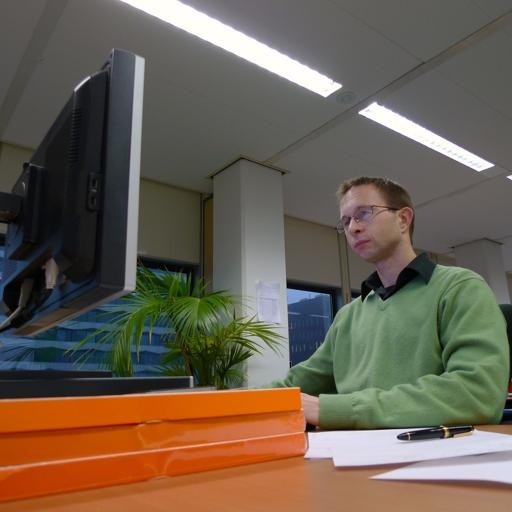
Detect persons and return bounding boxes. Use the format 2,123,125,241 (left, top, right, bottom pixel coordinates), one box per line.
253,175,510,429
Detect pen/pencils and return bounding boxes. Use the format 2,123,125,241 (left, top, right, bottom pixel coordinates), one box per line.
397,422,477,442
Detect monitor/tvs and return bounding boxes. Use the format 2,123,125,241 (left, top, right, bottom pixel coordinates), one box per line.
0,49,145,336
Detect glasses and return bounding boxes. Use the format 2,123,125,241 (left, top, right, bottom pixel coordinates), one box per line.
334,204,400,234
